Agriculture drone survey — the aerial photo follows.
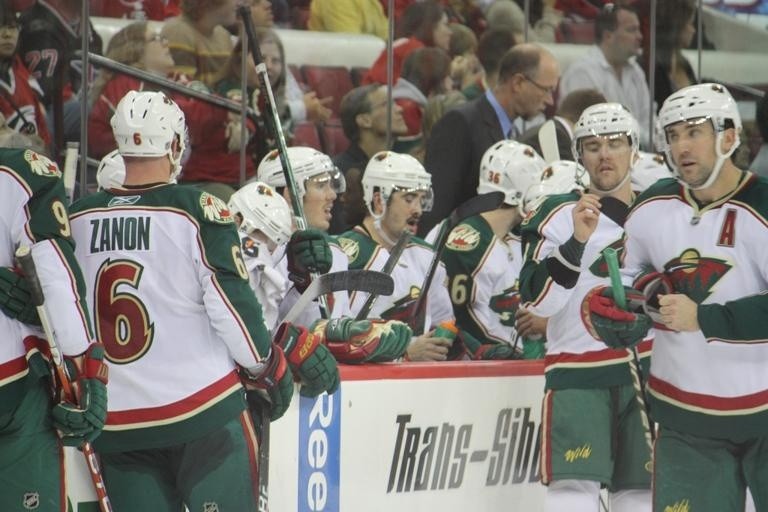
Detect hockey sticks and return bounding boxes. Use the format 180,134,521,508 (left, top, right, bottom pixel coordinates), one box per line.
273,270,393,343
407,192,506,328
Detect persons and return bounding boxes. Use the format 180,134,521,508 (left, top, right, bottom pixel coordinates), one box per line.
0,1,698,511
581,82,768,512
748,90,767,178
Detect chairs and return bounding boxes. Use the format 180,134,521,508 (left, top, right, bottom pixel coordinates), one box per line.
302,65,352,123
290,123,321,154
322,119,351,159
350,67,371,86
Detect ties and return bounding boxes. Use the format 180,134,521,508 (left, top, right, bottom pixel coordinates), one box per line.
508,128,518,139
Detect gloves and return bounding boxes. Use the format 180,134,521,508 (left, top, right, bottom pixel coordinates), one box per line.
234,342,294,421
580,283,655,350
309,316,414,366
472,343,524,360
273,321,341,397
632,265,675,325
286,229,333,302
51,342,108,446
0,266,41,326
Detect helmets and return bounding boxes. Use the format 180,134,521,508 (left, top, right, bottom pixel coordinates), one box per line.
476,139,547,206
362,150,434,218
109,89,186,158
95,149,182,194
227,182,292,247
630,151,676,192
656,82,742,158
538,160,590,195
256,146,347,194
573,102,640,157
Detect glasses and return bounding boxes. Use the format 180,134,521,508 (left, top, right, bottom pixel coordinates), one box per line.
146,34,168,44
511,71,553,93
1,22,22,33
603,3,614,15
